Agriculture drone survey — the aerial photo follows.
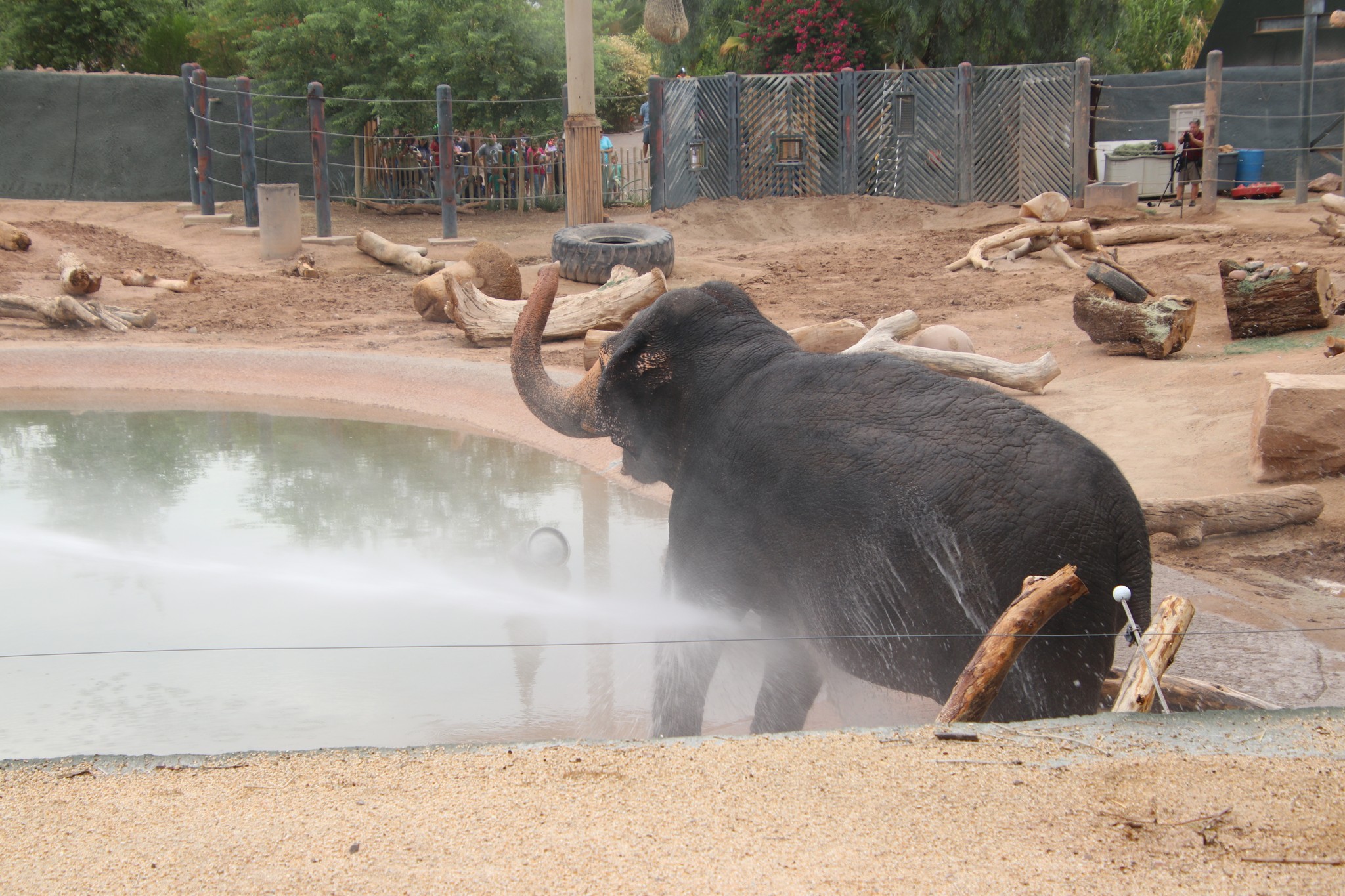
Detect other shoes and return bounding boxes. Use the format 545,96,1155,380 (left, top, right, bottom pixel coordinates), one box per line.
644,160,648,162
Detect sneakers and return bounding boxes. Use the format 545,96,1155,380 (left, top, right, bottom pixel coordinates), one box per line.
1170,199,1182,207
1189,201,1197,206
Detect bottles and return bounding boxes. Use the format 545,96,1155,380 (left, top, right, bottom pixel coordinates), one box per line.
1148,202,1157,207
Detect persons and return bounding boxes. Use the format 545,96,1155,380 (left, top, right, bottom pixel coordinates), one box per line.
1169,118,1204,206
639,94,650,162
674,67,691,78
598,127,623,203
377,128,567,208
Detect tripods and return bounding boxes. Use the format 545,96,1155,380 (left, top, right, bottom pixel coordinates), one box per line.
1158,144,1202,218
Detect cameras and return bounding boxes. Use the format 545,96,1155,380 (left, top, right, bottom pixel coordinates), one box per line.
1183,132,1191,143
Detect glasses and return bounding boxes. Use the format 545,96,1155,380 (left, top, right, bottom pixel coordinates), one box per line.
600,131,603,133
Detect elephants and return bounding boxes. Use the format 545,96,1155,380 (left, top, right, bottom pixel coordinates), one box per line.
509,259,1154,739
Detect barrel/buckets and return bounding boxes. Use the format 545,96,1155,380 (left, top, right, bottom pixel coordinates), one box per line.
1217,151,1237,190
1235,149,1264,188
